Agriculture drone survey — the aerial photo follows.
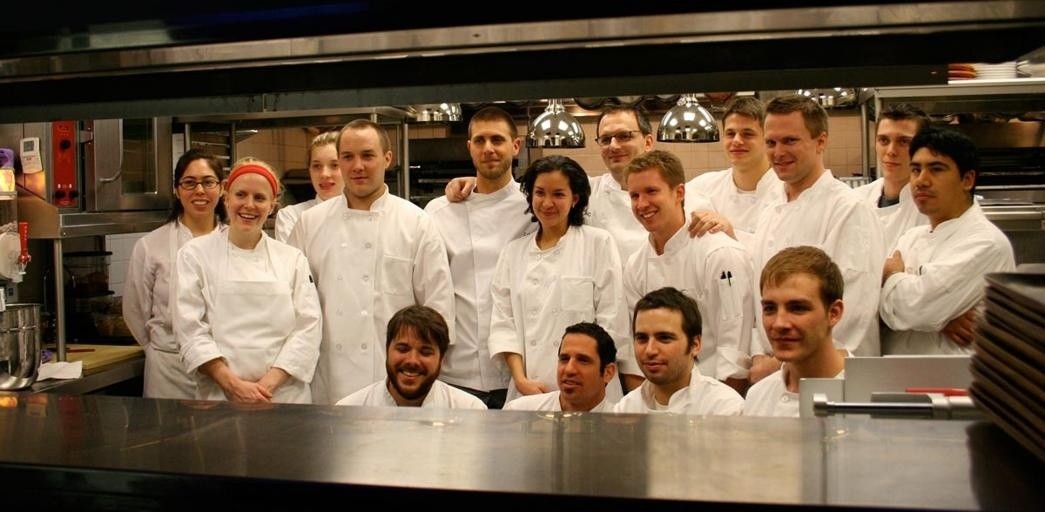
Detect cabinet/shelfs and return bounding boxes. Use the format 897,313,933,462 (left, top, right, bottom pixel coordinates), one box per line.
858,78,1044,263
0,106,416,394
1,1,1044,512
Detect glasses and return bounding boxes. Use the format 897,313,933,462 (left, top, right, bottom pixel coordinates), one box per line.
594,130,641,147
176,178,221,191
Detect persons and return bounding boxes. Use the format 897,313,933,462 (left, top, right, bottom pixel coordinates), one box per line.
335,306,489,409
848,103,930,262
879,120,1017,358
611,287,744,416
272,131,347,248
414,105,536,409
620,149,755,385
744,245,857,418
683,96,782,236
122,150,231,402
501,322,617,414
288,119,457,407
688,95,881,386
169,157,324,406
444,106,652,269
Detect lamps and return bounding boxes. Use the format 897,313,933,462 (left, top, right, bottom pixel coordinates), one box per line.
792,87,855,108
417,103,463,122
526,98,585,148
658,93,720,142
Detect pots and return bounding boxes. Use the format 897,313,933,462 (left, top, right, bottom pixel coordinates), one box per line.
0,303,43,391
